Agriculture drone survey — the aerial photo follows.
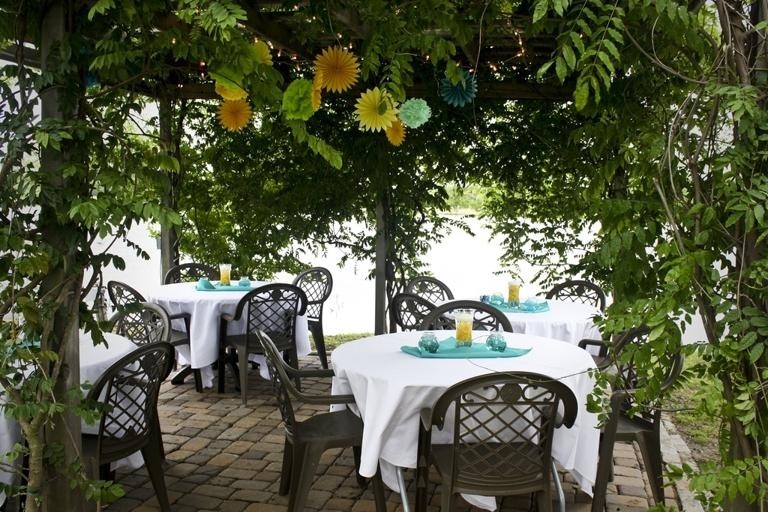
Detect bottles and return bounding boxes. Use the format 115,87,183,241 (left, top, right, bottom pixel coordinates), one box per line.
198,276,209,288
417,332,440,354
486,332,507,352
239,276,251,287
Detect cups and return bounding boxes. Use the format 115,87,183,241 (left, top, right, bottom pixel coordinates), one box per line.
491,295,504,305
453,308,476,347
219,263,231,287
508,281,520,307
480,295,489,303
